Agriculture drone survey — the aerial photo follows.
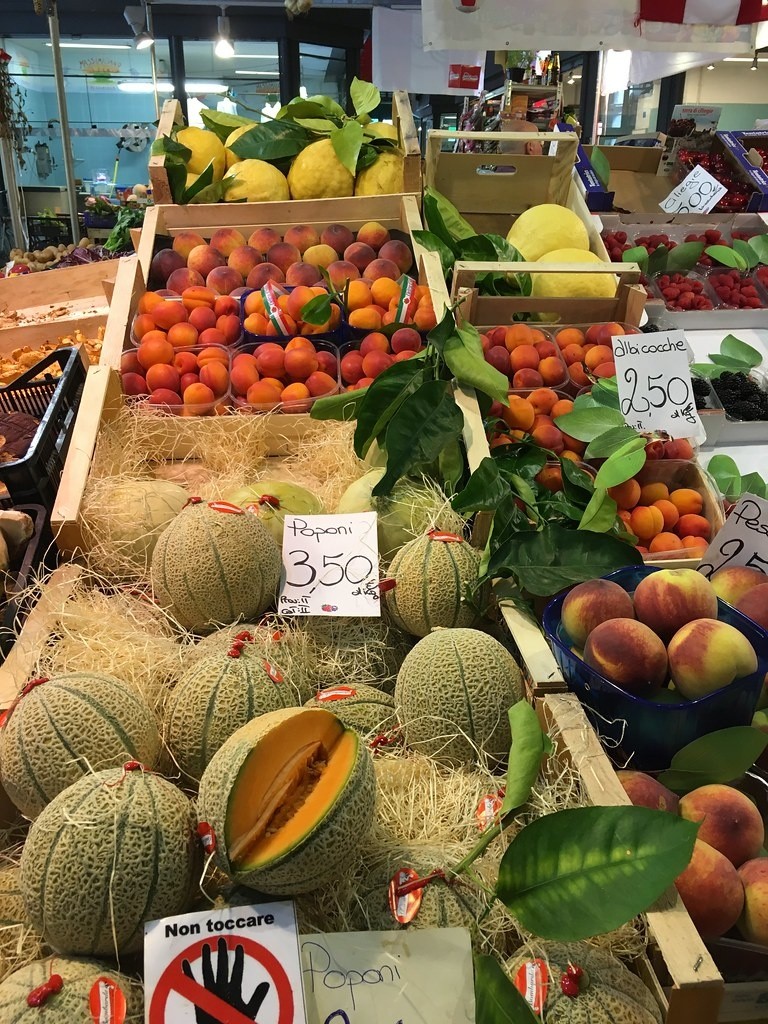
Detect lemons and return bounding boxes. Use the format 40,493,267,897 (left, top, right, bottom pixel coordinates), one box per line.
172,125,406,204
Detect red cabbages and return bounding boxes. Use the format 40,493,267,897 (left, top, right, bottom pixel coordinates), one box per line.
55,245,136,268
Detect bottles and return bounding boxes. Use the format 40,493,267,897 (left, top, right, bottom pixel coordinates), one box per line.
523,53,560,86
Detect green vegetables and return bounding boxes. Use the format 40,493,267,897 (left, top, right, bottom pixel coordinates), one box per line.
87,196,146,251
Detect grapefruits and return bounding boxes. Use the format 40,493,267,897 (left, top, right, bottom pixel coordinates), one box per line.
505,203,617,322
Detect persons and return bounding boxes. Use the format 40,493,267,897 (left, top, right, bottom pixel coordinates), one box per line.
494,119,542,174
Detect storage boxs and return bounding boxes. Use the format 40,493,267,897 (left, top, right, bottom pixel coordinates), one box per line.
29,224,70,238
85,212,117,229
419,129,767,985
0,346,87,667
120,229,428,415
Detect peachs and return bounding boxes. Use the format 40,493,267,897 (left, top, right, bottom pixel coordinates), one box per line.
119,222,440,418
476,322,713,562
560,564,768,949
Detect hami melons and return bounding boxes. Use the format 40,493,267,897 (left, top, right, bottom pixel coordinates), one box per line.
0,500,528,1024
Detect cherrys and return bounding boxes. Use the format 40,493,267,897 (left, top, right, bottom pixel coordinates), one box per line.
671,142,768,213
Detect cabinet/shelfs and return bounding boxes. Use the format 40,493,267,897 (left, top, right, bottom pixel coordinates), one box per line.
461,74,566,130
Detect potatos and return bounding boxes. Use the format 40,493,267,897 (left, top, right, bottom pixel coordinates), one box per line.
9,236,93,272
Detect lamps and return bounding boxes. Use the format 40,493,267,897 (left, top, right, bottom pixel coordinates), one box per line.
750,52,759,72
123,5,155,50
215,15,234,58
567,67,575,85
707,63,715,70
